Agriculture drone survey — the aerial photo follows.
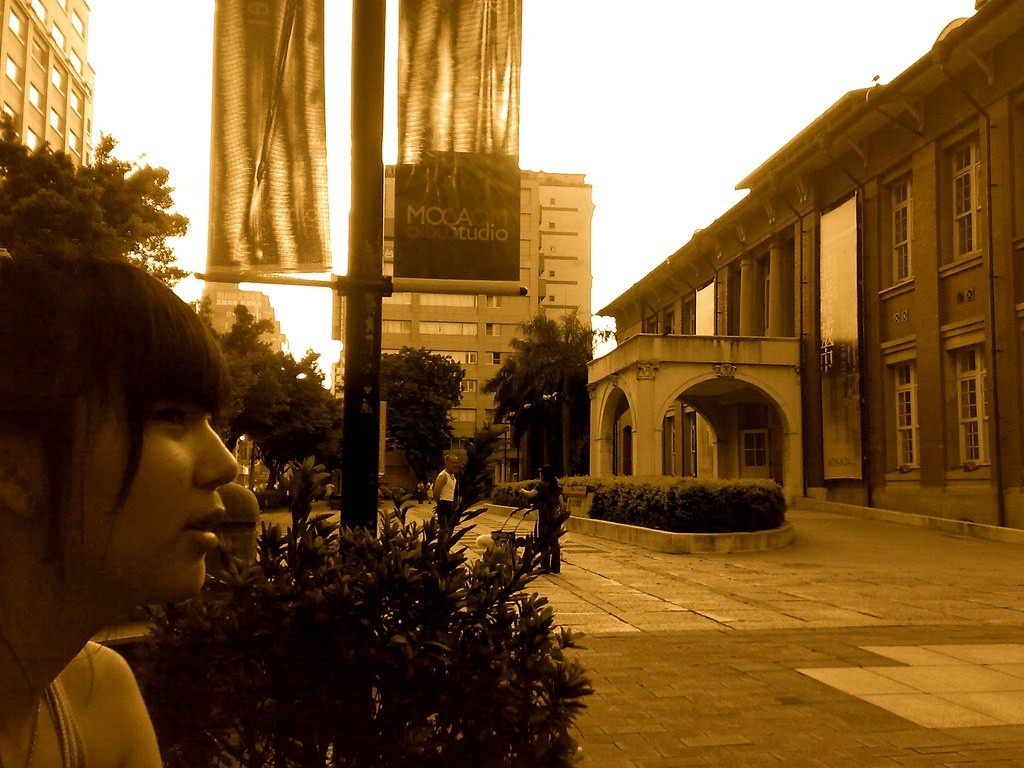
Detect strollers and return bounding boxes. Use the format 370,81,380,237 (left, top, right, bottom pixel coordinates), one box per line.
491,505,533,548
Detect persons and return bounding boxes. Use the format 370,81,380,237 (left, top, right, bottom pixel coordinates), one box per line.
519,464,564,574
425,480,434,504
433,455,460,552
416,479,425,504
0,256,238,768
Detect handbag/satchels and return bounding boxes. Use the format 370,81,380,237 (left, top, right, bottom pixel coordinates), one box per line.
419,484,424,490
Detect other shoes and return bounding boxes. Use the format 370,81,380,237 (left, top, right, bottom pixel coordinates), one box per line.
536,567,561,575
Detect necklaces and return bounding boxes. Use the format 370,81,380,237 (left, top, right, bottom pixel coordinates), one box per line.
24,704,40,768
